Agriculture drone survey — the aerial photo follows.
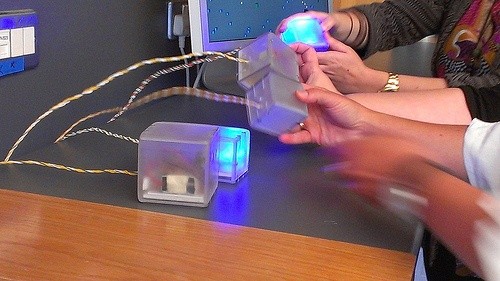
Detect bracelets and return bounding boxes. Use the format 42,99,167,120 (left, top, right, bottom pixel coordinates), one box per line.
337,10,353,43
346,8,367,49
377,71,400,92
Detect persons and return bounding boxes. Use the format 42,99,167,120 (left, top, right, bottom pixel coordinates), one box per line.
288,41,499,125
279,87,498,281
274,1,500,91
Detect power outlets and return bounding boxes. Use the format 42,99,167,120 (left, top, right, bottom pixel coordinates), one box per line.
1,11,41,78
168,2,189,41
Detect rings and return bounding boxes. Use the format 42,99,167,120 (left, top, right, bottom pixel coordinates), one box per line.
299,64,304,67
298,122,306,132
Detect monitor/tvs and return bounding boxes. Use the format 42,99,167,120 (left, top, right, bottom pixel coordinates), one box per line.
187,0,334,55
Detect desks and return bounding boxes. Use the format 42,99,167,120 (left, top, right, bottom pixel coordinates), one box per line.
1,41,429,281
